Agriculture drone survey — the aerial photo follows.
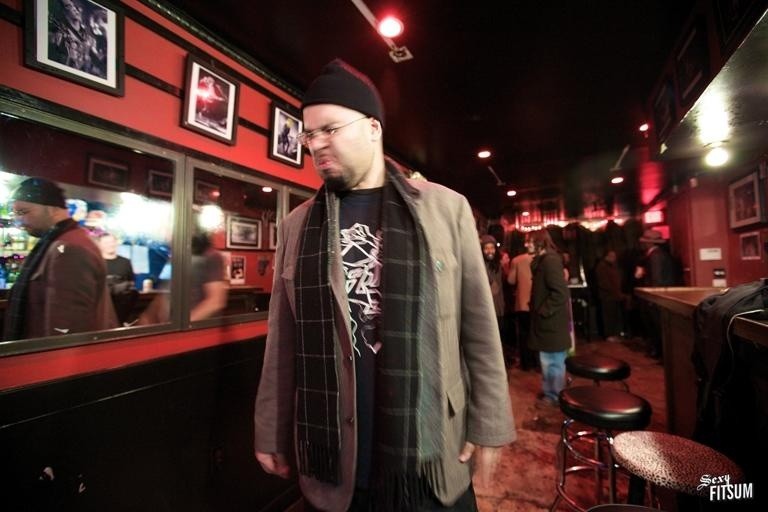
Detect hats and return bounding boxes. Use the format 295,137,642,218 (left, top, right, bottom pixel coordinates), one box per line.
480,235,498,246
639,229,668,245
12,178,66,209
302,59,386,131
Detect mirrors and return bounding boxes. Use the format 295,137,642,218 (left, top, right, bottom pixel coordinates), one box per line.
182,152,284,331
0,84,181,361
285,183,314,222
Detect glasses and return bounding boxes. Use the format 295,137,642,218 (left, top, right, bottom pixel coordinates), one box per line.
297,117,372,148
10,204,38,220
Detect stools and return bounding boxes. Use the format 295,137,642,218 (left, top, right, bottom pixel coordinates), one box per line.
549,385,652,512
564,352,631,446
587,431,742,512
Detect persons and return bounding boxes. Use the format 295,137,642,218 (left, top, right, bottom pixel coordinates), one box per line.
498,252,519,357
479,235,507,348
2,178,120,340
594,251,628,341
504,253,537,367
545,225,576,387
255,57,516,511
132,221,230,325
524,227,572,406
637,232,670,357
96,234,138,322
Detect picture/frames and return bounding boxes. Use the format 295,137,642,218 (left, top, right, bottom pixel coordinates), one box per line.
180,51,241,146
23,0,125,98
146,166,173,199
268,220,277,250
651,80,676,140
670,36,708,107
226,215,262,249
268,99,305,169
193,177,223,204
740,231,761,260
86,151,132,192
726,168,762,232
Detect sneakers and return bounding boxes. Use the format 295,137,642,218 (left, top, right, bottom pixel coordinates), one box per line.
536,396,558,413
